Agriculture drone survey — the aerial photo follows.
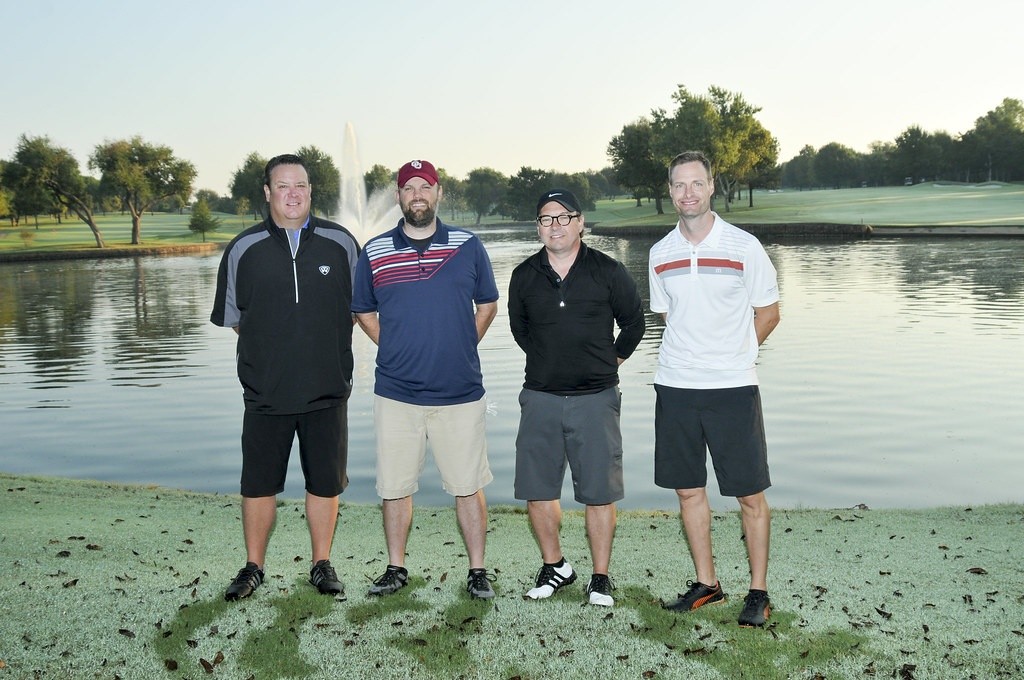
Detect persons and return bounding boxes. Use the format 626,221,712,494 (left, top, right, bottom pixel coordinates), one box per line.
350,160,500,598
208,154,362,602
649,151,781,629
508,189,647,608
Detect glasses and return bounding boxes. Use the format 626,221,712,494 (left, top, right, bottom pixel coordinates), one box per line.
536,215,581,227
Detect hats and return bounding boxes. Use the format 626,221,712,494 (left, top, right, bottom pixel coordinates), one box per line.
536,189,581,216
398,160,439,188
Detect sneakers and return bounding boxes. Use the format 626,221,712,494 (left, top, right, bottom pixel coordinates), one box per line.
225,562,265,602
368,565,408,596
467,568,500,601
308,560,344,595
587,574,618,607
738,589,774,628
663,580,724,613
522,556,577,601
